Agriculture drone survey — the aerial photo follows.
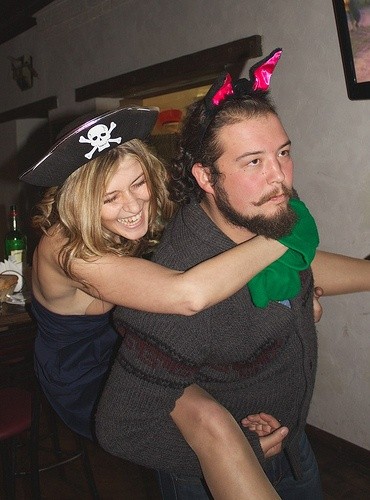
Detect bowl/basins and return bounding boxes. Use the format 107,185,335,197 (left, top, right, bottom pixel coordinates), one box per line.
0,274,19,302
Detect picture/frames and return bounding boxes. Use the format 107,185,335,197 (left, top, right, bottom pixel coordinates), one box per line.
332,0,370,101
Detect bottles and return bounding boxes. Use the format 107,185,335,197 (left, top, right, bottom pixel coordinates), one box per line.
4,204,28,263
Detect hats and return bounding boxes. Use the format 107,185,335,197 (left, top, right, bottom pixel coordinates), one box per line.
18,104,159,187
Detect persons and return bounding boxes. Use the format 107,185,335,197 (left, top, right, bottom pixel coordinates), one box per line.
94,47,324,500
18,104,370,500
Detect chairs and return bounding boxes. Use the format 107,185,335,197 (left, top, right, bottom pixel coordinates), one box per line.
0,318,99,500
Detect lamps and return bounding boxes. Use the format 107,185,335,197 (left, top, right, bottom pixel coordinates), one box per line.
9,54,40,92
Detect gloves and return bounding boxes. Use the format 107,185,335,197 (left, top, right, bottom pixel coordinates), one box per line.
248,198,319,307
